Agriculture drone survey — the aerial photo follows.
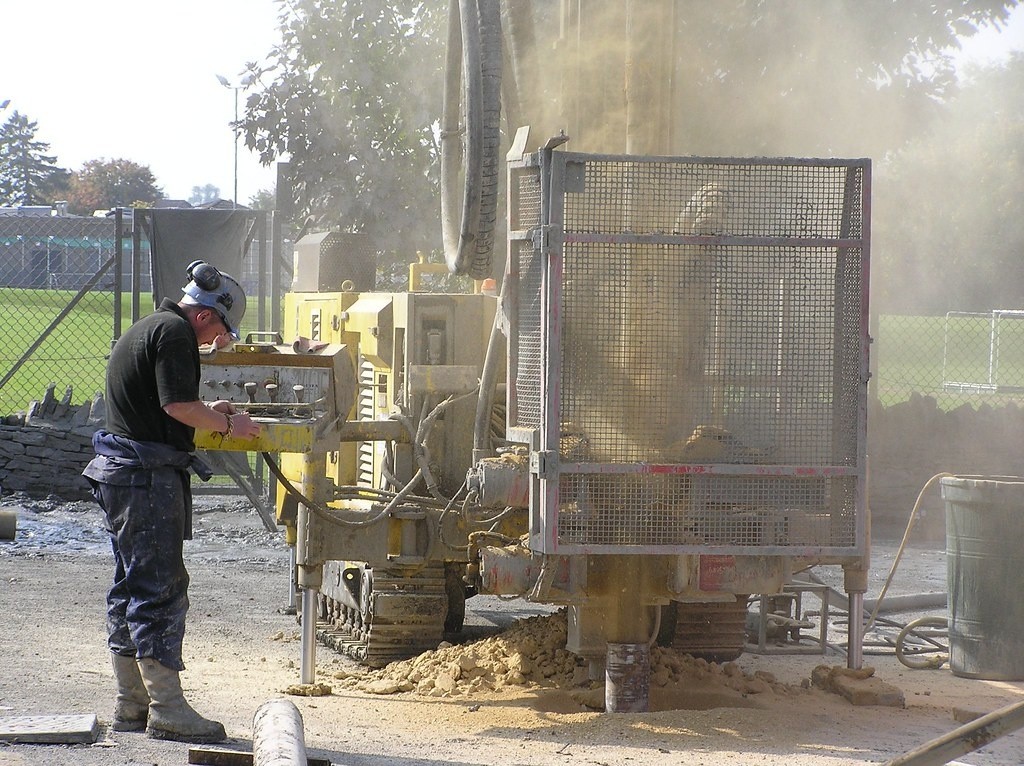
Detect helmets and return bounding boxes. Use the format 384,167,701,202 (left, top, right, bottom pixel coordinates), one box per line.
180,260,246,341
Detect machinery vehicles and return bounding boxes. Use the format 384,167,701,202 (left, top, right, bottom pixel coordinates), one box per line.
194,4,875,714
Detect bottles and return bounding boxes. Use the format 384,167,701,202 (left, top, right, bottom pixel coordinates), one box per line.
480,277,497,297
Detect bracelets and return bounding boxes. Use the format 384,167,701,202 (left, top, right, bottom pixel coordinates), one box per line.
209,413,234,448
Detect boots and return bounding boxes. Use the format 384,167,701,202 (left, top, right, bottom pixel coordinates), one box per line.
136,657,227,745
110,651,153,732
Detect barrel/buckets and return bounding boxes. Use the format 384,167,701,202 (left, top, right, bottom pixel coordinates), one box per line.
939,474,1024,680
606,643,650,713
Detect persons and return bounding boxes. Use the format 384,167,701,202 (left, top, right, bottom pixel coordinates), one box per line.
81,260,263,744
